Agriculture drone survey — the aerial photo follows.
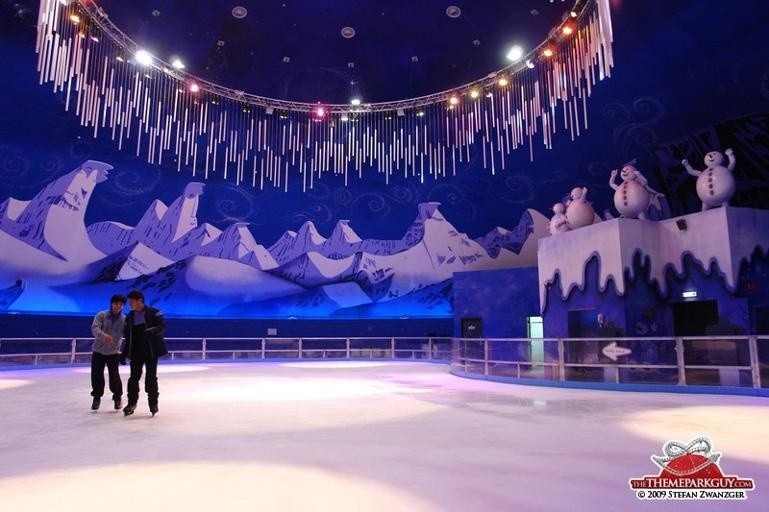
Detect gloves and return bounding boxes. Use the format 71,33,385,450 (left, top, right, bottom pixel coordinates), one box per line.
143,331,150,341
120,354,126,365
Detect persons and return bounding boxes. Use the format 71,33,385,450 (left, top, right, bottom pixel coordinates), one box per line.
90,295,128,413
120,291,167,417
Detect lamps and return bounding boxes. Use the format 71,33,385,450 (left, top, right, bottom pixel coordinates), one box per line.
71,2,580,122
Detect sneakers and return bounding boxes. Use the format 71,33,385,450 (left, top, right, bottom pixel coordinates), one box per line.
92,398,100,409
150,400,157,412
115,400,121,409
124,404,136,411
